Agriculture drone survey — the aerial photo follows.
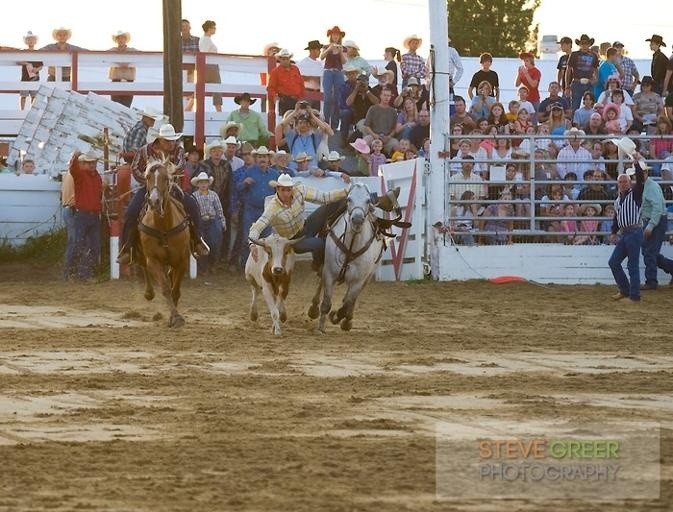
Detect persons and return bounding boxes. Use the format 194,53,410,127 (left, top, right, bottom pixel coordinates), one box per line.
60,19,673,302
16,31,44,111
37,25,90,103
21,160,37,175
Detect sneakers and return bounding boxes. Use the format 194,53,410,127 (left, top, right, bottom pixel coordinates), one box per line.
194,242,208,257
375,186,400,211
117,253,131,263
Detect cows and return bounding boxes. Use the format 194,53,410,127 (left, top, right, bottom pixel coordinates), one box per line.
244,233,308,341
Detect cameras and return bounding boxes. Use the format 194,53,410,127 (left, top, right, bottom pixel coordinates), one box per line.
299,102,309,109
332,43,342,48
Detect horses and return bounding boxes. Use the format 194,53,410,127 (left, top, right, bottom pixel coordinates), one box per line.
307,179,385,334
134,149,195,329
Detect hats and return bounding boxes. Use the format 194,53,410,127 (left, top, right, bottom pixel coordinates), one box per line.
23,31,37,45
556,37,573,44
627,160,651,175
343,40,360,50
637,77,656,85
552,102,563,109
269,173,299,187
519,53,534,60
220,122,243,136
645,35,666,47
611,137,637,159
575,34,595,46
52,27,71,41
341,66,394,84
136,107,161,120
613,41,624,48
205,136,346,166
77,151,99,161
349,138,371,154
603,104,620,120
564,128,586,144
152,123,183,141
404,34,422,49
112,30,131,43
605,75,622,88
304,40,323,50
327,26,344,37
408,77,418,86
517,85,529,93
265,43,293,61
234,93,256,106
191,172,214,187
